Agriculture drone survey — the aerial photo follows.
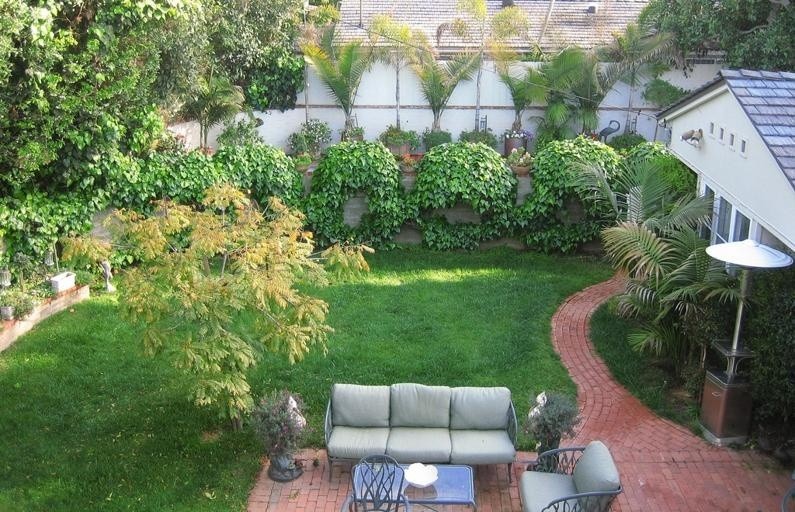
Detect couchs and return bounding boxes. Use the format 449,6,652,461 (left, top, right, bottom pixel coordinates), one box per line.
322,382,518,484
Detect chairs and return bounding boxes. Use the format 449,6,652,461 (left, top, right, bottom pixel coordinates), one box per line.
341,453,411,512
518,440,622,511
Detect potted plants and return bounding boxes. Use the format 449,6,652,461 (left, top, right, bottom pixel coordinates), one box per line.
526,392,581,472
253,389,315,482
